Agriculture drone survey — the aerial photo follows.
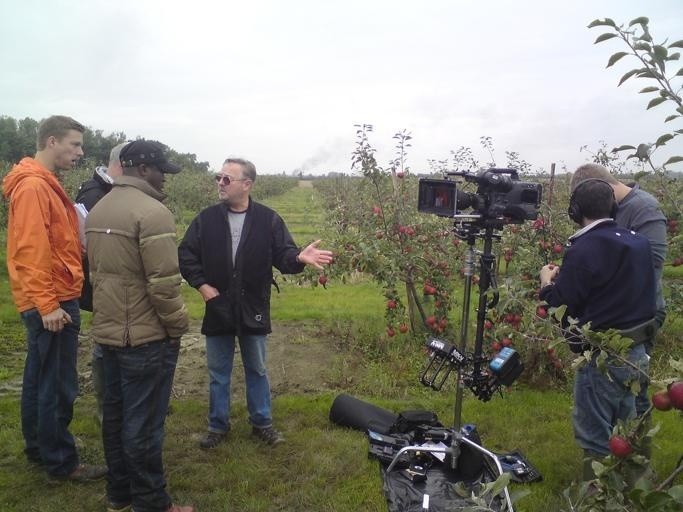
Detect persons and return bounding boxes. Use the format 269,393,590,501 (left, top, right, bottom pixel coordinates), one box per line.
73,142,131,311
571,163,667,328
539,179,658,505
84,140,193,512
177,158,334,449
1,116,109,487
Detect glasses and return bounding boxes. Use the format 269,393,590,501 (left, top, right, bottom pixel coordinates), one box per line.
214,175,247,185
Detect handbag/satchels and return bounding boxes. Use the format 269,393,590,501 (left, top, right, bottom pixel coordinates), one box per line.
389,409,444,435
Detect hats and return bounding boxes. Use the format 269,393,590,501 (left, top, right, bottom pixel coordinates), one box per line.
118,138,182,174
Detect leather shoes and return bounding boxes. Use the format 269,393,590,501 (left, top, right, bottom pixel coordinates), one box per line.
69,461,109,482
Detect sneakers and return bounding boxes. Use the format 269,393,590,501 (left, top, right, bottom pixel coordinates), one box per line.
166,505,193,512
198,420,232,450
105,498,133,512
251,424,287,449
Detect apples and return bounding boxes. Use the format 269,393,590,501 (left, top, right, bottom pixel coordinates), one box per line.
610,434,635,455
651,380,683,412
319,172,683,372
